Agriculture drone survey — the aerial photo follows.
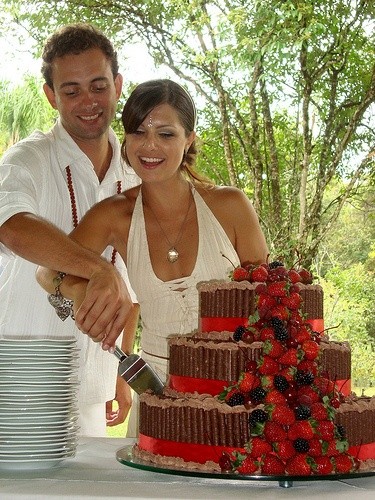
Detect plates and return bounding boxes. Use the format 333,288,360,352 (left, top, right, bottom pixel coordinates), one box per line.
0,334,80,472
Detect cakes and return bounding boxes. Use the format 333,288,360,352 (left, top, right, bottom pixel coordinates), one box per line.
132,251,375,474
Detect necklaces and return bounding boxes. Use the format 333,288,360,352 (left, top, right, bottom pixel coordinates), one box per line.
144,186,191,263
66,165,122,266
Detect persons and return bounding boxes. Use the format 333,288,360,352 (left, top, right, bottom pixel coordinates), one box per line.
1,23,143,441
37,79,270,440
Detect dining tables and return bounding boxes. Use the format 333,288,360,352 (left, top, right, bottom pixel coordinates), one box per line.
0,435,375,500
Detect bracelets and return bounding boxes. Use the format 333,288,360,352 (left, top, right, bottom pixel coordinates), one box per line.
47,273,77,322
